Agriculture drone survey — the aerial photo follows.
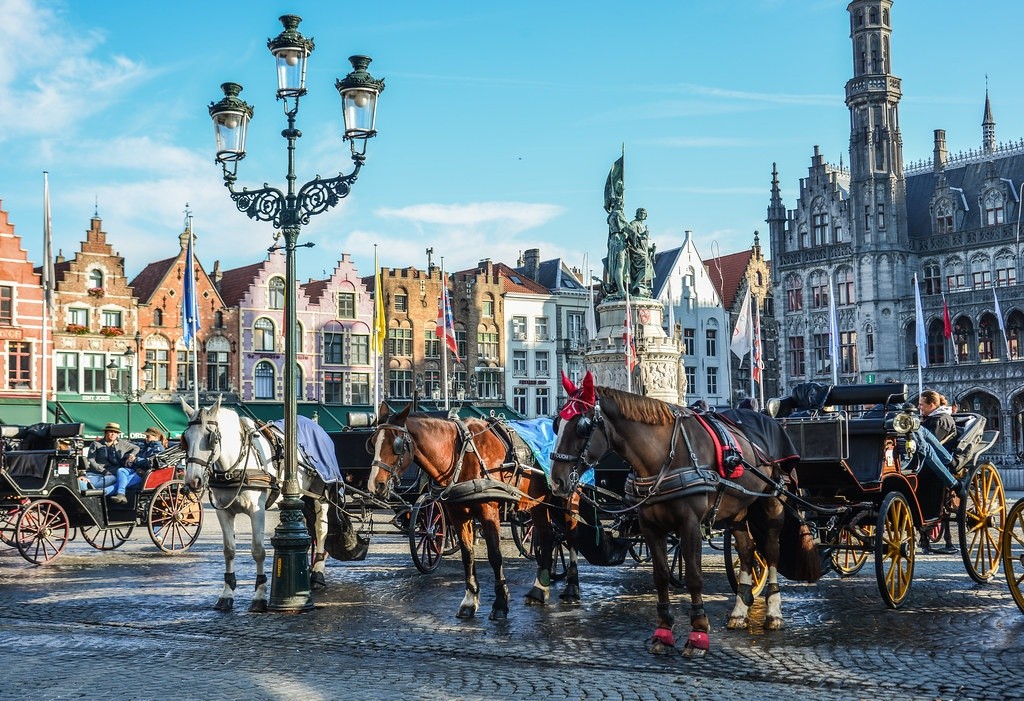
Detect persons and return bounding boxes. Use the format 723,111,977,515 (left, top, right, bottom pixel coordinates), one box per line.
606,198,657,297
862,389,971,499
76,422,168,503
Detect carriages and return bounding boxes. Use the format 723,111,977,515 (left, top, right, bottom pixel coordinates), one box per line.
364,371,1008,659
178,391,545,571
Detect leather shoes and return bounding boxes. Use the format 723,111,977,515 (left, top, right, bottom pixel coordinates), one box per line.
949,443,973,473
956,471,970,498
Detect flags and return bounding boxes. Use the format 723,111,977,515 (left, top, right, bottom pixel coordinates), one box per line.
622,298,637,375
730,286,763,383
436,273,461,363
995,293,1004,331
42,188,55,315
943,299,953,339
371,257,387,356
182,230,202,349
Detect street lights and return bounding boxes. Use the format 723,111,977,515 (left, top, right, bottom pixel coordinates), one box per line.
106,345,153,437
205,13,386,610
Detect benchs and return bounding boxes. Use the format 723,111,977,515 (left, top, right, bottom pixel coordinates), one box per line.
324,429,382,472
774,384,909,436
4,423,86,478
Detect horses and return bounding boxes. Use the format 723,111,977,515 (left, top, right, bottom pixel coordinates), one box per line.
547,386,821,658
365,400,583,620
177,392,346,613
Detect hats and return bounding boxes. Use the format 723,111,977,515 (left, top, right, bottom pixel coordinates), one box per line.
142,427,161,437
102,422,121,433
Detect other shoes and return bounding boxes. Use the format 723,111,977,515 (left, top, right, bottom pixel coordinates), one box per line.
111,494,128,504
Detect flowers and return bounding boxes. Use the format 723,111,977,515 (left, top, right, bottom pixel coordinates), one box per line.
100,326,124,336
87,287,104,298
66,323,91,334
